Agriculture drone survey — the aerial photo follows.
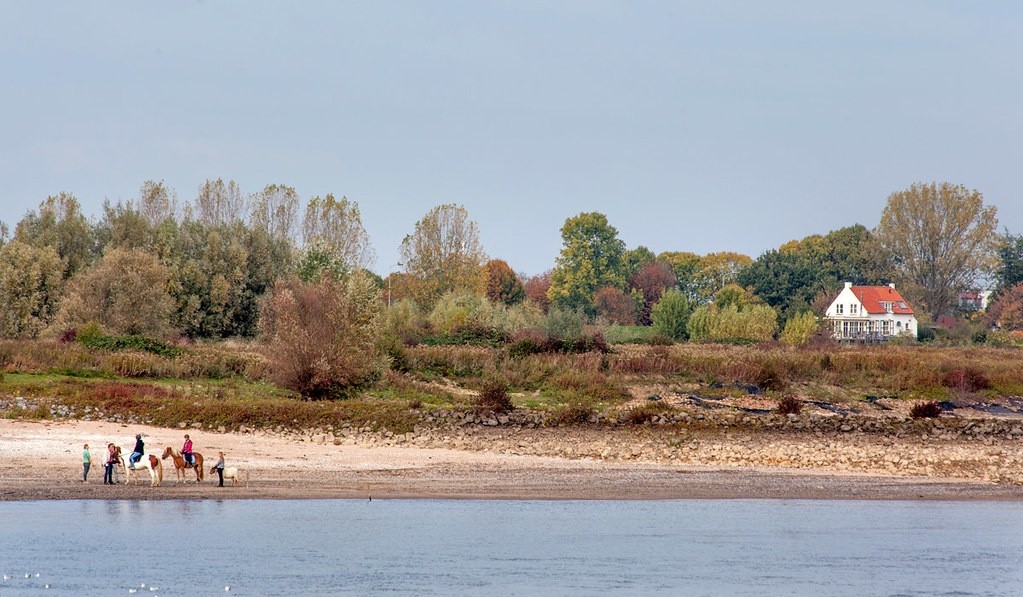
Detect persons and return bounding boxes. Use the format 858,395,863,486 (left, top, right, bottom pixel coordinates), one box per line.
82,444,92,484
102,442,120,485
127,435,144,470
214,452,225,487
180,434,193,468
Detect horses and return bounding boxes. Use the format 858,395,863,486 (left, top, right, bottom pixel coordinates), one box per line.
162,445,204,483
113,452,162,487
